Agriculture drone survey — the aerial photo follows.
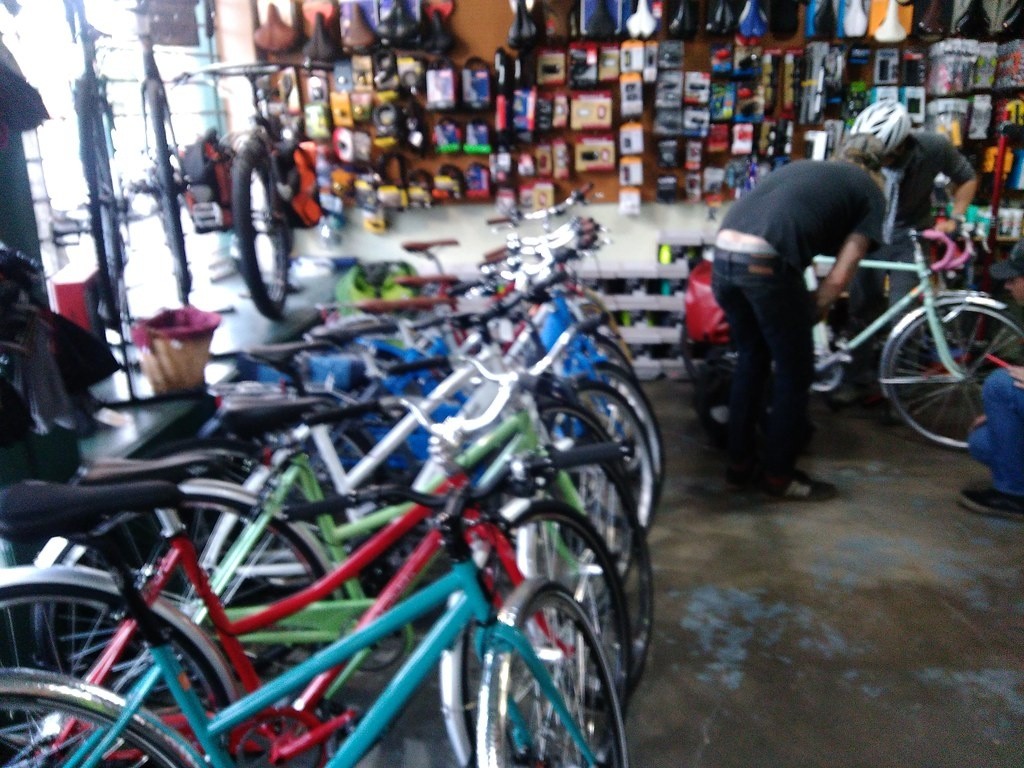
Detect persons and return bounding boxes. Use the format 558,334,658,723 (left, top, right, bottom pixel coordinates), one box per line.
850,101,981,413
712,153,887,500
959,241,1024,518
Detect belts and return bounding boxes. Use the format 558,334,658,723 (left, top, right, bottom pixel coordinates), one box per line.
712,250,783,271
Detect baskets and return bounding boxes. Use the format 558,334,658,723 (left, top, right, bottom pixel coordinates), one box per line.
131,307,220,398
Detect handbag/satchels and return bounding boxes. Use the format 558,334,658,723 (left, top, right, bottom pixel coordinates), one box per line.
271,140,322,227
684,256,731,343
179,129,229,235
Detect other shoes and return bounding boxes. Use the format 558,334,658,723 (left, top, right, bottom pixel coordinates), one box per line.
757,470,838,503
962,488,1024,521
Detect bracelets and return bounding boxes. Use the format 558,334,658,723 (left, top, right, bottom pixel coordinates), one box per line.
951,215,966,223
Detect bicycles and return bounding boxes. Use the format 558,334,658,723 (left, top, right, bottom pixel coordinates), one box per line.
171,56,336,321
0,179,1023,768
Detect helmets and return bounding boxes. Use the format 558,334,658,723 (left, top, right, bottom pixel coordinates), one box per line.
852,96,913,159
